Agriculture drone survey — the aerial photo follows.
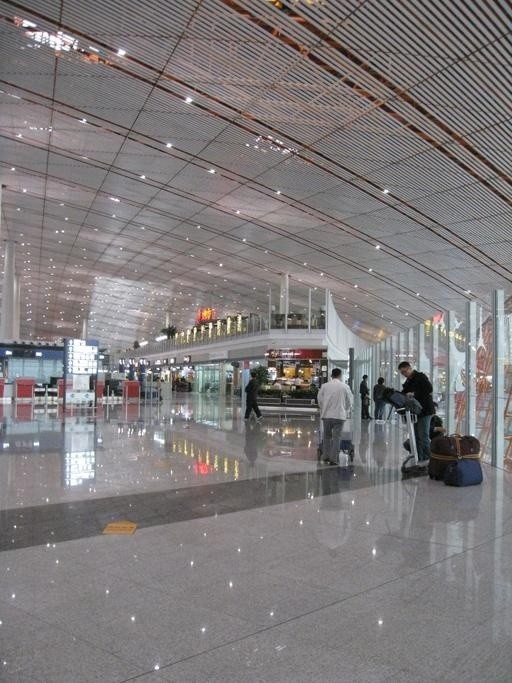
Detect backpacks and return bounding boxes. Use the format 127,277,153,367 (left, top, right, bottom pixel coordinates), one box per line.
382,385,423,415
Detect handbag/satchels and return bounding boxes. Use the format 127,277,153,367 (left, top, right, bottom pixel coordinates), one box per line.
428,433,483,486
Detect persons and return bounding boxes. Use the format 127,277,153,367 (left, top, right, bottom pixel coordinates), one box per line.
399,362,446,460
374,377,386,419
317,369,353,466
244,372,263,423
360,375,373,419
291,375,304,392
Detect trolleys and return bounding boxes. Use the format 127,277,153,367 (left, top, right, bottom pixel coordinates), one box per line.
316,403,355,466
